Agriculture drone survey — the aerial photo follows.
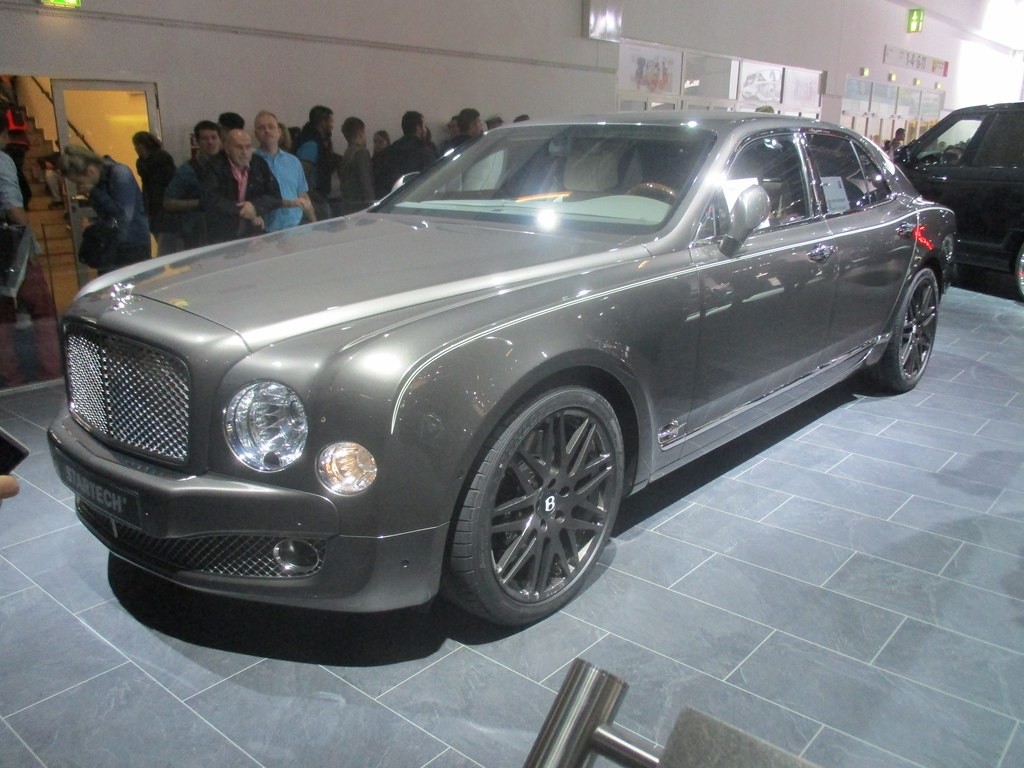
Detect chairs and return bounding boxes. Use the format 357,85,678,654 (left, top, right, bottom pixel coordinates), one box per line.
757,177,796,229
561,146,619,194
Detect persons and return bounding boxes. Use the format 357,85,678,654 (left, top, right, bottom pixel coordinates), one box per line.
59,145,152,277
36,139,69,209
278,104,440,221
0,76,62,501
442,107,530,192
132,131,188,258
162,111,316,243
883,128,905,151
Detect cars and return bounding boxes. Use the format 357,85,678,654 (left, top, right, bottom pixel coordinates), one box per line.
894,102,1024,300
46,107,955,626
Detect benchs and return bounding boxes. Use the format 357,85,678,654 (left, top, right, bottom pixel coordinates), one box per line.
792,176,875,218
